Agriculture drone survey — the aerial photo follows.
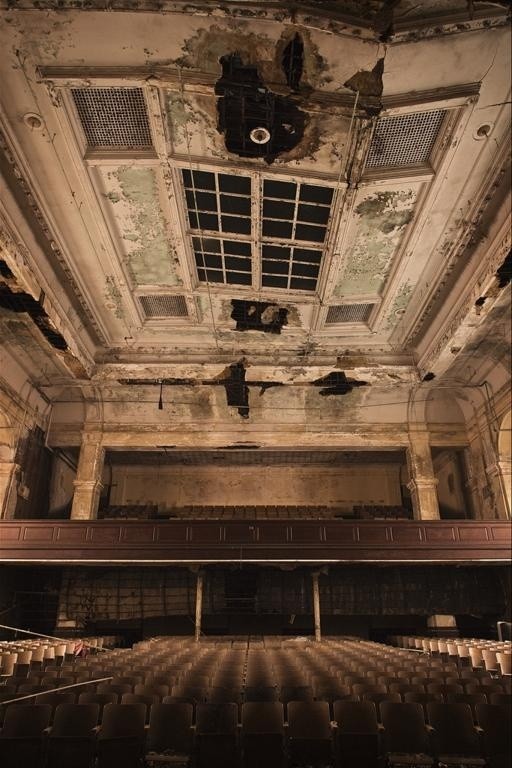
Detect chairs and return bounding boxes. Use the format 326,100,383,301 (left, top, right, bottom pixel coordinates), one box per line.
1,633,510,767
